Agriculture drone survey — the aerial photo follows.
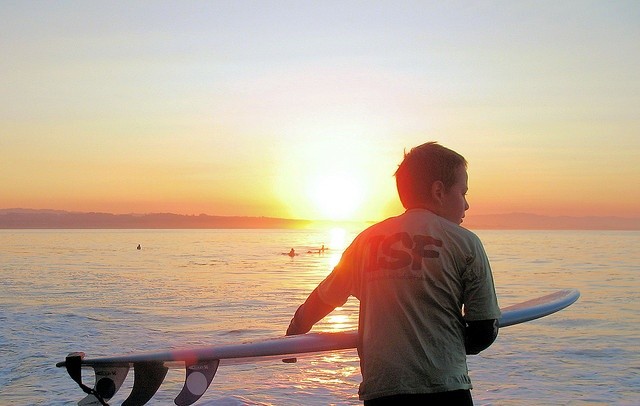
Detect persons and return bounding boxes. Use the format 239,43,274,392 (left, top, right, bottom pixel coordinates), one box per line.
282,141,502,406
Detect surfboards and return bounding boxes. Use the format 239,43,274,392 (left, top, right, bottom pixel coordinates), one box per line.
53,286,583,405
